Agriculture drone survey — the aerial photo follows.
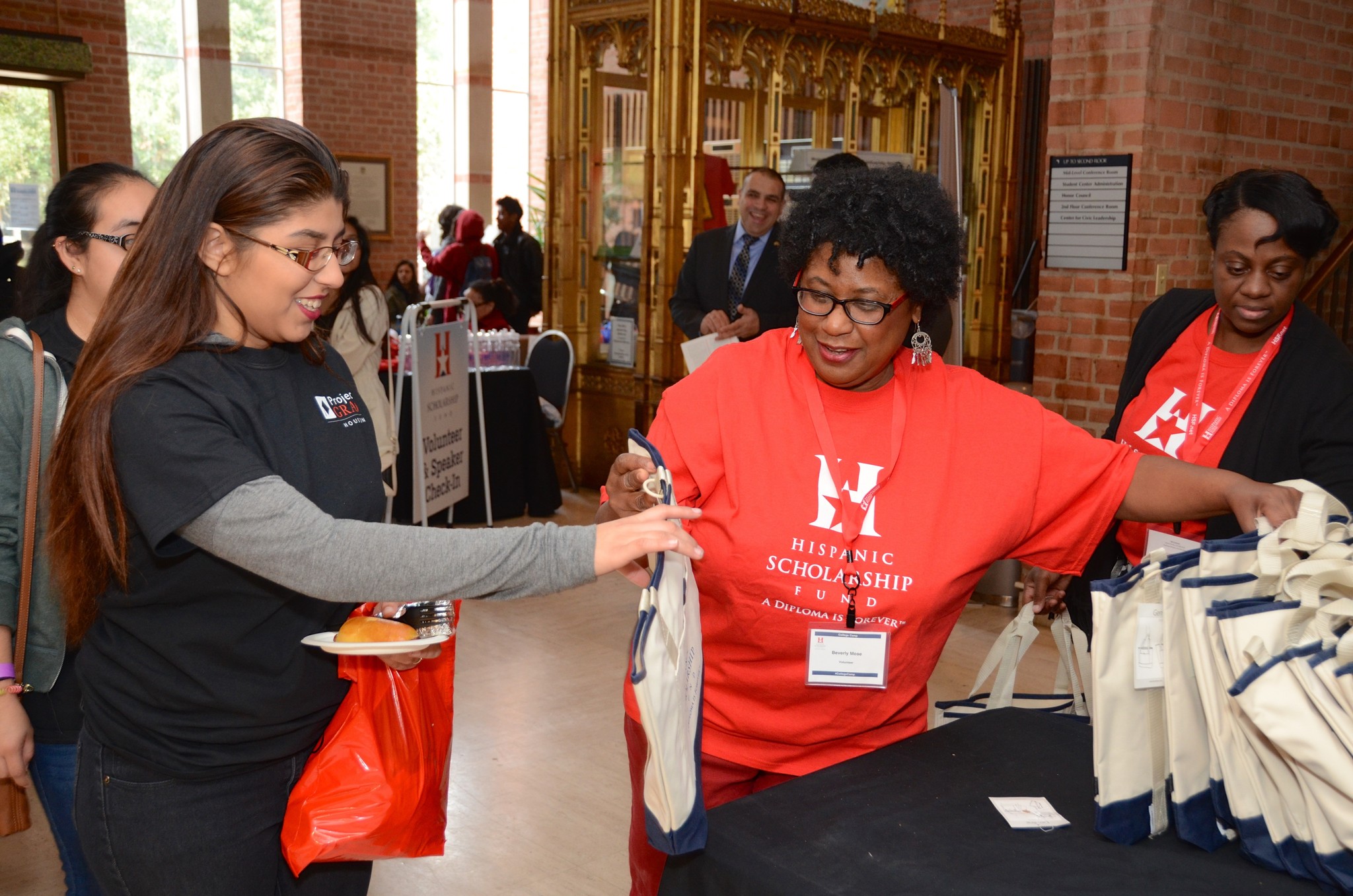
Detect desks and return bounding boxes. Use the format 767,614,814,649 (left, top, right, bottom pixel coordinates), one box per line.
376,367,563,526
657,706,1331,895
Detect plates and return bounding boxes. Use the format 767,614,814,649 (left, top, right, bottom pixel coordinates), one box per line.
301,630,451,655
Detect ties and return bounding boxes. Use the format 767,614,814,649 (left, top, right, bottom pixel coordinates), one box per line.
726,234,760,324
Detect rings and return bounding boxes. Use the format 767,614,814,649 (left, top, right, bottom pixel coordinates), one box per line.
710,314,717,320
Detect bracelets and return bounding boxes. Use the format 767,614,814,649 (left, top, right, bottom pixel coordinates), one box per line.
0,683,24,696
0,663,15,680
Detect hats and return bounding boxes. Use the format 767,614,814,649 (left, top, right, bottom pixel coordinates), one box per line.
439,205,463,230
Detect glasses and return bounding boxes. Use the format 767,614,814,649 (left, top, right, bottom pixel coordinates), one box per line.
78,230,137,253
461,301,489,311
221,226,360,272
791,268,908,325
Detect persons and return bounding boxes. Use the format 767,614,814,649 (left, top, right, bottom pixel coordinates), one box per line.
384,257,422,335
598,165,1305,895
658,167,808,377
415,199,499,331
0,162,173,895
451,278,521,350
486,194,544,340
40,116,709,895
321,214,400,510
1021,163,1351,653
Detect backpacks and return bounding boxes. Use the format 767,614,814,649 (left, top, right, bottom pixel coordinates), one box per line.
452,244,492,300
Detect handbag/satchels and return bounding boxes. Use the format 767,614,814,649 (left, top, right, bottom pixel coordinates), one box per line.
934,601,1093,729
281,597,463,880
1089,478,1353,896
0,773,33,840
627,428,710,855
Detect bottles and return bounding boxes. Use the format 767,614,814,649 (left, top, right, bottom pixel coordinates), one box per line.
390,314,523,372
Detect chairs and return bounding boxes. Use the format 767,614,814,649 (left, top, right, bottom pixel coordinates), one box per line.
525,327,580,495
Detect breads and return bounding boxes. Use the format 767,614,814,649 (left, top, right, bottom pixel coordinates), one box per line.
335,615,421,643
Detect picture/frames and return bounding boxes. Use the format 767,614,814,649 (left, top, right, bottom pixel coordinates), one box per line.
333,152,397,242
